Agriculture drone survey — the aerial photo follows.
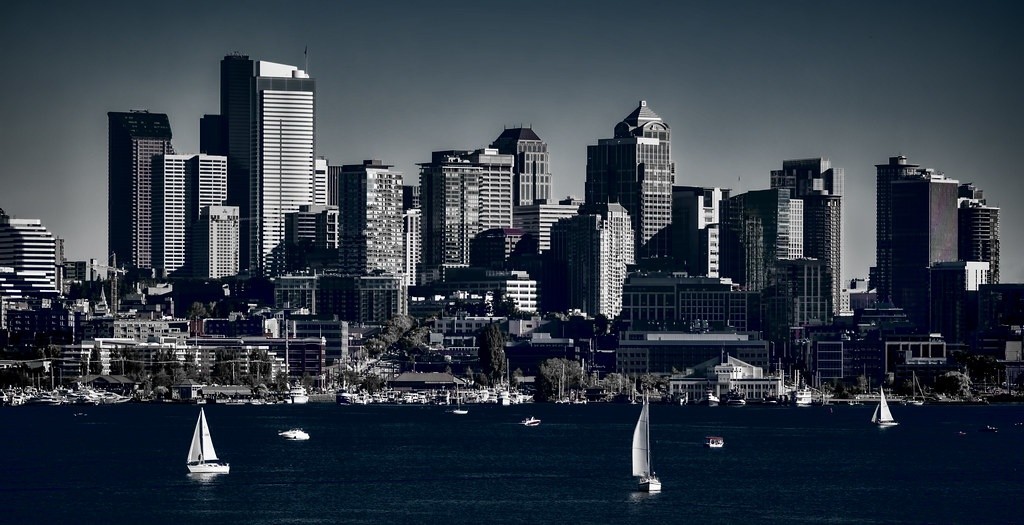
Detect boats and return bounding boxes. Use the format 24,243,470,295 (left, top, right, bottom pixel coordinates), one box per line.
703,437,724,448
518,416,541,426
795,381,864,407
278,428,310,440
0,379,533,406
628,382,663,404
695,379,779,406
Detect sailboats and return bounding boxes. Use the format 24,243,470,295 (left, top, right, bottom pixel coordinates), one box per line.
871,386,900,428
444,383,468,415
632,388,662,494
554,358,587,404
186,407,230,474
912,370,926,406
